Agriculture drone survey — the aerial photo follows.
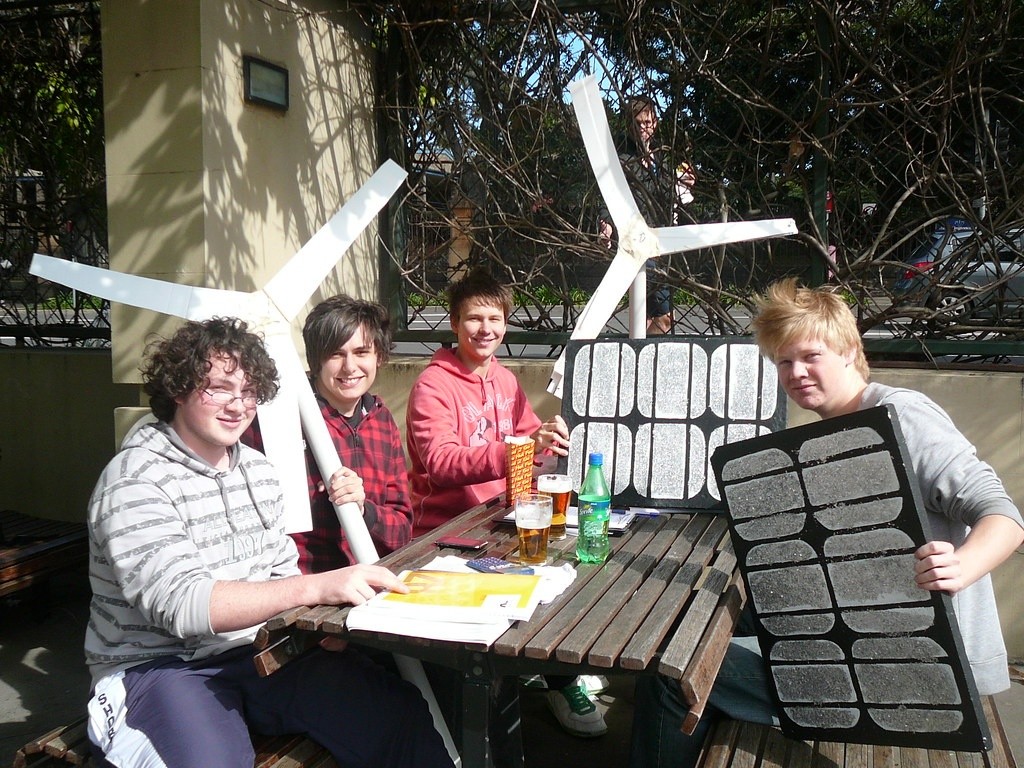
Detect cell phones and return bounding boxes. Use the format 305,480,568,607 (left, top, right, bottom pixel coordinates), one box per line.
434,535,489,550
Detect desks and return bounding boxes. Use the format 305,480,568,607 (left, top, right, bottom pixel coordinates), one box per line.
251,487,750,768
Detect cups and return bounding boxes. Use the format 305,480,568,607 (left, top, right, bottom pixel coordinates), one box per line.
536,474,573,540
514,493,553,566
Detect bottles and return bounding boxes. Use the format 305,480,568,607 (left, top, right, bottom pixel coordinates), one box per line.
575,453,611,563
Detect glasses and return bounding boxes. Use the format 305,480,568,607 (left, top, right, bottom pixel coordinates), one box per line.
199,386,262,408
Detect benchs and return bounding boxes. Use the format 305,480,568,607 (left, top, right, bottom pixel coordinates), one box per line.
693,695,1018,768
11,695,341,768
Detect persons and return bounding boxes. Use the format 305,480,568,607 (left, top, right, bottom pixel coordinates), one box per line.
642,276,1024,764
596,94,696,334
406,263,609,738
83,294,415,767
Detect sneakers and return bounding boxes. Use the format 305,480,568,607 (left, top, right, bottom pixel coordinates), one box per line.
545,680,608,736
519,675,609,695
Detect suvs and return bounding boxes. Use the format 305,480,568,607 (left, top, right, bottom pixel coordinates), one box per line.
894,228,1024,327
